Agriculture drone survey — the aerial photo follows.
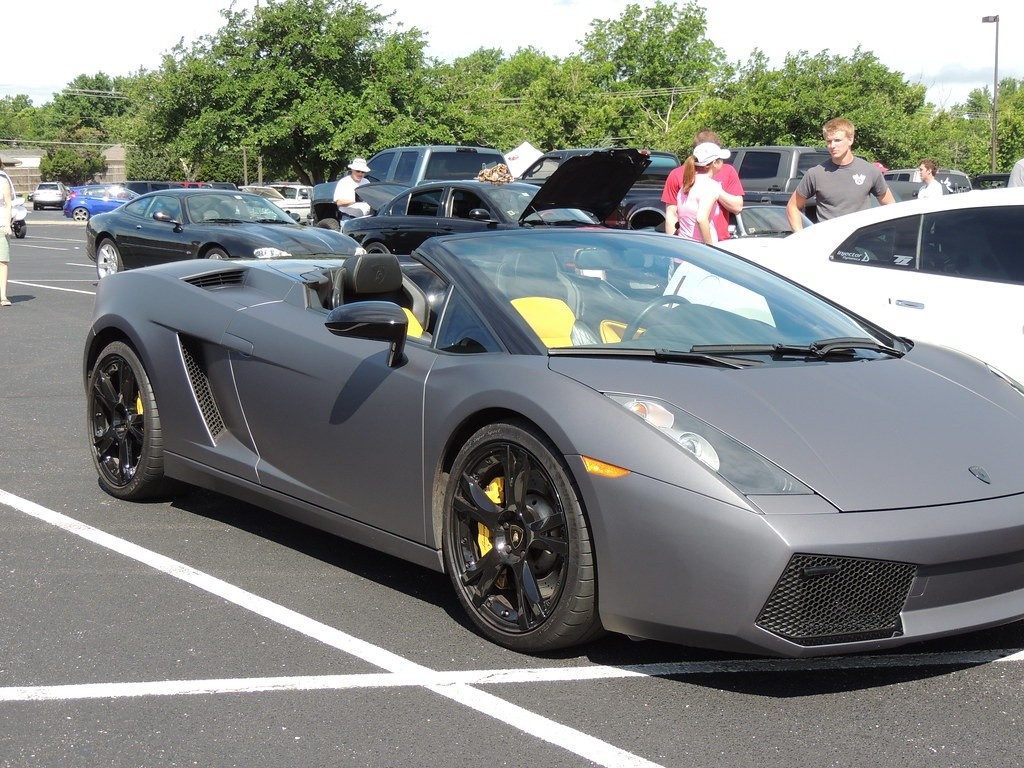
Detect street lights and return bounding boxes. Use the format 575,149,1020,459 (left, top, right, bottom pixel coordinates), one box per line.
983,15,1000,175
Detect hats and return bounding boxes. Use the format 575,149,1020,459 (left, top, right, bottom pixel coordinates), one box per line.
872,163,889,173
347,158,371,172
693,142,731,167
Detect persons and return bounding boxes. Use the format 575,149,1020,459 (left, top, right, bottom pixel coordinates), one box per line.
786,117,896,234
0,158,16,306
1007,159,1024,188
872,163,889,176
917,159,942,198
333,158,371,227
674,142,731,273
661,129,746,282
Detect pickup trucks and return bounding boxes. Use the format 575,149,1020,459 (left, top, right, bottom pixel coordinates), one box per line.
515,150,804,242
314,145,538,239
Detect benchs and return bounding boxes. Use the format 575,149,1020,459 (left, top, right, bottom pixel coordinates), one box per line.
924,218,1010,281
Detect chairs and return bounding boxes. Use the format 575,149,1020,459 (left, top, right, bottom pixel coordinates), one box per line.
329,254,429,333
496,249,584,321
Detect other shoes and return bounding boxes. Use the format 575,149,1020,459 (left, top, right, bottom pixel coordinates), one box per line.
1,300,12,306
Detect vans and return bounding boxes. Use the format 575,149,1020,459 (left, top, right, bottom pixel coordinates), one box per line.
883,169,972,195
725,147,833,196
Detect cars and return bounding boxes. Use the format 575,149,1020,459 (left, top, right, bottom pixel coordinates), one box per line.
64,184,143,221
662,186,1023,390
85,188,367,281
342,147,652,256
240,184,314,224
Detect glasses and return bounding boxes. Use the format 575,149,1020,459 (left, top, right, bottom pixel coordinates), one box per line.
356,171,364,173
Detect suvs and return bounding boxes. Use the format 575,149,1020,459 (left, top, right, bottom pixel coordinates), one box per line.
32,180,68,210
121,181,238,204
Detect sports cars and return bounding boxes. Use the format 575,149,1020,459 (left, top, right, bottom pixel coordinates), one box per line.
83,228,1024,660
